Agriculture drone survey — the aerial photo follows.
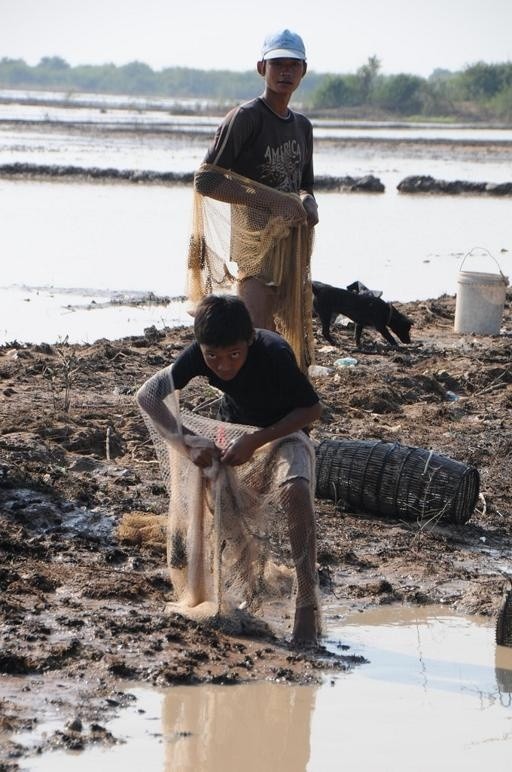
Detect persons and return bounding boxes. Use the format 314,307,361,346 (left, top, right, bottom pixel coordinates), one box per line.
194,28,319,335
135,292,322,649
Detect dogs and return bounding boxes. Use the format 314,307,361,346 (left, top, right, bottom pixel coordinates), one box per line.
312,279,415,348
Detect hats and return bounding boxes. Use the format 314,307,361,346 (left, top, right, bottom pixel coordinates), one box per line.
261,28,307,61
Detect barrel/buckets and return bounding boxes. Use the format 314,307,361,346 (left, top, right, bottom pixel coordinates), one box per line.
453,247,509,336
